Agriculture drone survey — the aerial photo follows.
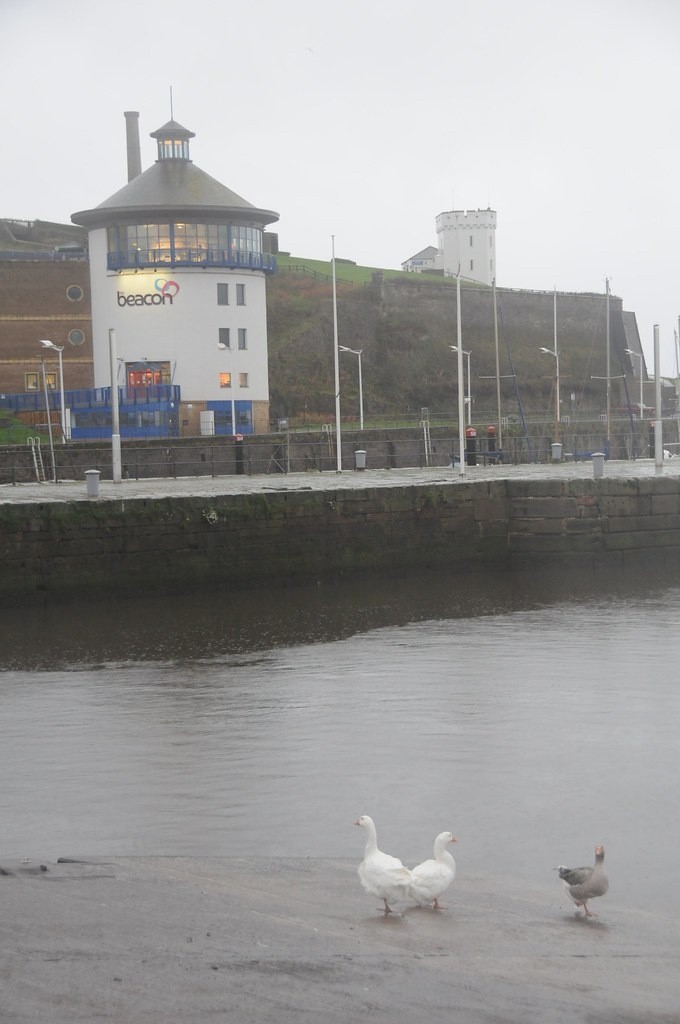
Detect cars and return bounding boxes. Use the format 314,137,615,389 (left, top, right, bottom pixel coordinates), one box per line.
617,403,656,414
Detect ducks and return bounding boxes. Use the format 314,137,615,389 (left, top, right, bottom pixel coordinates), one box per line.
354,816,412,912
407,831,458,909
555,845,609,917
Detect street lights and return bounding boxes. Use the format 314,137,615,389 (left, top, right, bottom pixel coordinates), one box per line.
217,342,236,436
38,340,65,444
339,345,363,431
539,347,559,422
449,346,472,425
624,349,642,419
